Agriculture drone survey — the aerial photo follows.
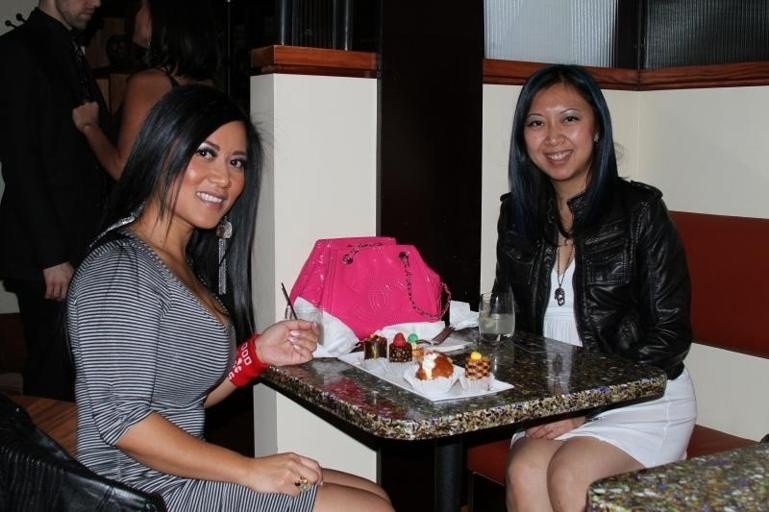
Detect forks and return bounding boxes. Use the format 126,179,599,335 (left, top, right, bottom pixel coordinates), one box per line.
416,325,457,346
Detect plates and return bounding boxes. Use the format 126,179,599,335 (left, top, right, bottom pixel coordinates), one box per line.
335,351,515,404
450,311,479,332
428,337,473,352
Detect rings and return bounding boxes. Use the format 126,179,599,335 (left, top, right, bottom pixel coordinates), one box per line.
295,478,309,492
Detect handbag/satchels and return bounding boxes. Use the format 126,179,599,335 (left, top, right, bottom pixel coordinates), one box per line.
286,238,445,359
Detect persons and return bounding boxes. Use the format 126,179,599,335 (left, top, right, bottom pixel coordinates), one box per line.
75,1,217,247
64,84,393,511
1,0,106,399
491,65,697,512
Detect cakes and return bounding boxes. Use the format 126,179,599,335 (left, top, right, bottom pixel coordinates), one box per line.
363,333,491,380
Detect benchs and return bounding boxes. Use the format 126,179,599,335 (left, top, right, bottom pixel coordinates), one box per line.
460,208,769,511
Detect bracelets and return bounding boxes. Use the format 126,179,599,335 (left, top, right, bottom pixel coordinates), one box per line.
226,334,268,389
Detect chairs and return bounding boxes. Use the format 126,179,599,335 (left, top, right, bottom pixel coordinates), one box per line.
0,393,168,512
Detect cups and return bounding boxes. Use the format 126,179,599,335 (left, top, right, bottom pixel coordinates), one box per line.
287,305,324,348
481,342,516,378
478,292,516,341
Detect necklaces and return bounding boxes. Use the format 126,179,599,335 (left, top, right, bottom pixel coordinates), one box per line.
555,231,574,306
